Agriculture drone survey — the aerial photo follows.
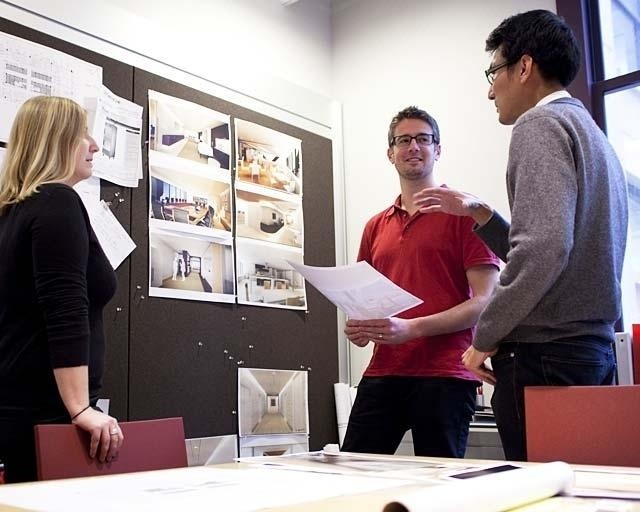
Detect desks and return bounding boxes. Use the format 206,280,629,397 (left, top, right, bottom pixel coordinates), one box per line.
0,449,640,512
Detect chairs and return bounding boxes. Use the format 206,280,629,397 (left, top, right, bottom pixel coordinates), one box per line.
523,384,640,466
32,415,190,480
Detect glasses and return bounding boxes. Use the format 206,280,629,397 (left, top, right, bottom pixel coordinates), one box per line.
485,60,509,84
390,133,439,147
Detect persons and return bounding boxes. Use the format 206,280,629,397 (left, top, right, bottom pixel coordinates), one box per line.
339,108,500,459
411,8,630,461
0,95,125,482
172,257,186,281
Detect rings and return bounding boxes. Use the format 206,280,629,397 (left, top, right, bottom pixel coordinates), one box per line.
378,334,383,340
110,429,119,435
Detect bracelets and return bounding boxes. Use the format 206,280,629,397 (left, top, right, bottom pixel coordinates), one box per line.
71,404,91,420
